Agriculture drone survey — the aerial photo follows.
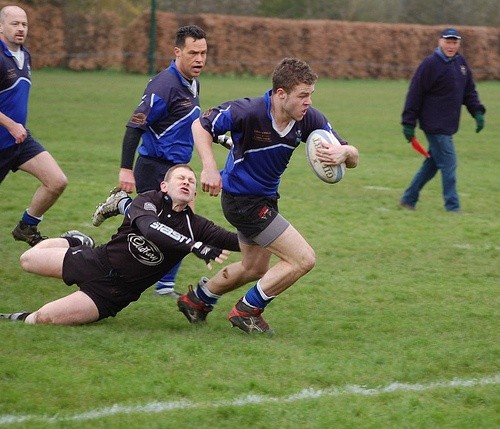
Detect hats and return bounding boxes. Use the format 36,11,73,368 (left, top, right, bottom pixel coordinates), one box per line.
440,27,462,39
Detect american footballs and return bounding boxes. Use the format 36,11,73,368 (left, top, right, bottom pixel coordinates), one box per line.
306,128,347,185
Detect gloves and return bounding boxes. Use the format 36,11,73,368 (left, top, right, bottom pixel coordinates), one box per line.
402,124,415,142
475,112,484,132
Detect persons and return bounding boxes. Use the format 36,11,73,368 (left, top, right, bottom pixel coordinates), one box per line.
0,4,68,247
0,164,240,326
178,58,358,335
400,28,486,213
94,25,206,294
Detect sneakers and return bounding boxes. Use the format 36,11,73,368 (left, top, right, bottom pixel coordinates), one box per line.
227,296,274,340
156,291,183,299
177,285,214,327
60,229,95,248
0,311,28,320
12,220,48,247
92,186,131,226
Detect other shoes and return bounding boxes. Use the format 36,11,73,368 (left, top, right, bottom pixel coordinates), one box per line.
399,200,416,211
